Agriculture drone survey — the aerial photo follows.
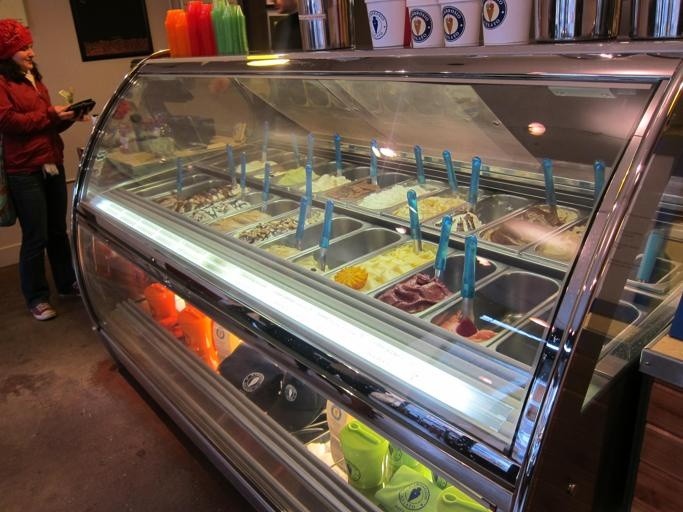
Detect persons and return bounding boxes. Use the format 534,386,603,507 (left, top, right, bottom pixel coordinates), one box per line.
0,19,92,322
265,0,298,14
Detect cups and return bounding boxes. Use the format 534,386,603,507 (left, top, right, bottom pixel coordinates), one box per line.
364,0,532,48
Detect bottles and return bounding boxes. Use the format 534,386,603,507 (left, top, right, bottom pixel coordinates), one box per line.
165,1,249,57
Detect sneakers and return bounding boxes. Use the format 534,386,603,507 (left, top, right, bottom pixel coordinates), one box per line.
29,301,57,321
59,280,81,296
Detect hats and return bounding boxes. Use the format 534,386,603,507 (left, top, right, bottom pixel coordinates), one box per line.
0,18,33,60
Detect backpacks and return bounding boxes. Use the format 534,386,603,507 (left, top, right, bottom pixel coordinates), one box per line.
0,172,17,228
